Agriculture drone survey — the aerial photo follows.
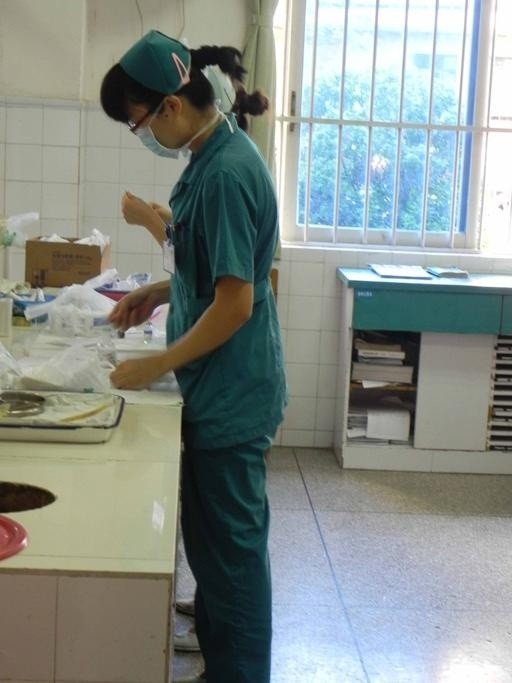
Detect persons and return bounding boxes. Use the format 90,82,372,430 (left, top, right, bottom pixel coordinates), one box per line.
118,65,281,658
95,25,290,682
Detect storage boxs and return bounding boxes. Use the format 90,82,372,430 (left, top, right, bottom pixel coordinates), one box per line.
25,236,110,289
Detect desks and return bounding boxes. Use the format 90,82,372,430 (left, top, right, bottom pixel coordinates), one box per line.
0,282,182,683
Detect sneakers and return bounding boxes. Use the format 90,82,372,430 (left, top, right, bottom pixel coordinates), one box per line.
172,669,206,682
175,597,197,617
173,626,201,652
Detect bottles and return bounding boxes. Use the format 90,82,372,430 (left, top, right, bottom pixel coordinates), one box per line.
144,323,153,344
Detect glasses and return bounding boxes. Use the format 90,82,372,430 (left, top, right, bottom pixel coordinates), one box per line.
125,93,167,136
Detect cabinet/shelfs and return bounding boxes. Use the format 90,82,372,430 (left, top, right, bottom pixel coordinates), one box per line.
333,267,512,475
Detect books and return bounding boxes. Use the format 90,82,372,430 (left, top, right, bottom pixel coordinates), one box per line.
347,329,417,442
367,261,433,279
424,265,470,279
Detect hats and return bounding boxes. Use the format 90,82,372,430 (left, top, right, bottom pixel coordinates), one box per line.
198,63,237,115
120,29,192,96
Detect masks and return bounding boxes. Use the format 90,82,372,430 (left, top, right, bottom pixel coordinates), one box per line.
133,95,235,160
